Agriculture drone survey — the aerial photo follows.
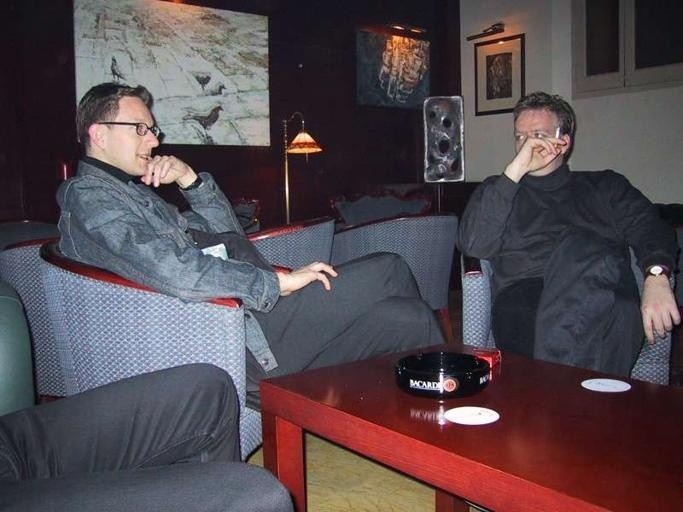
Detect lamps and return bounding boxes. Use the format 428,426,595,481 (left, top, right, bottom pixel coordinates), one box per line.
280,113,325,225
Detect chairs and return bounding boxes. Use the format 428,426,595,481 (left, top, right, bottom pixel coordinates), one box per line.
37,239,291,464
461,247,678,388
231,194,264,234
0,237,67,408
324,211,461,342
328,190,432,228
244,217,336,279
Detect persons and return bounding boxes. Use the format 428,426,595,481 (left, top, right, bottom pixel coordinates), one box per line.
54,79,446,417
456,89,682,383
1,362,297,510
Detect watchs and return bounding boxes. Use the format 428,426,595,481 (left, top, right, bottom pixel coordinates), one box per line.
642,265,670,281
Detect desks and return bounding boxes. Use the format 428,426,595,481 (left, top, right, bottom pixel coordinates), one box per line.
257,340,683,510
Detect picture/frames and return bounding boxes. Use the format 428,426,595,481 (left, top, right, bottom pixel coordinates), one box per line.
473,34,528,116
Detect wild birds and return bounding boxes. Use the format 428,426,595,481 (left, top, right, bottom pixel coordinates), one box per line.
111,56,126,82
197,84,226,96
194,75,211,91
183,106,223,131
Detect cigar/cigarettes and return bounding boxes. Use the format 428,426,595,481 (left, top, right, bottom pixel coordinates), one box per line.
554,124,560,149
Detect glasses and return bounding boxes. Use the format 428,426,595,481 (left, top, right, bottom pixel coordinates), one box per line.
99,122,160,137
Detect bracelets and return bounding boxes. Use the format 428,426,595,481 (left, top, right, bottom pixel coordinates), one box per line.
183,176,203,191
